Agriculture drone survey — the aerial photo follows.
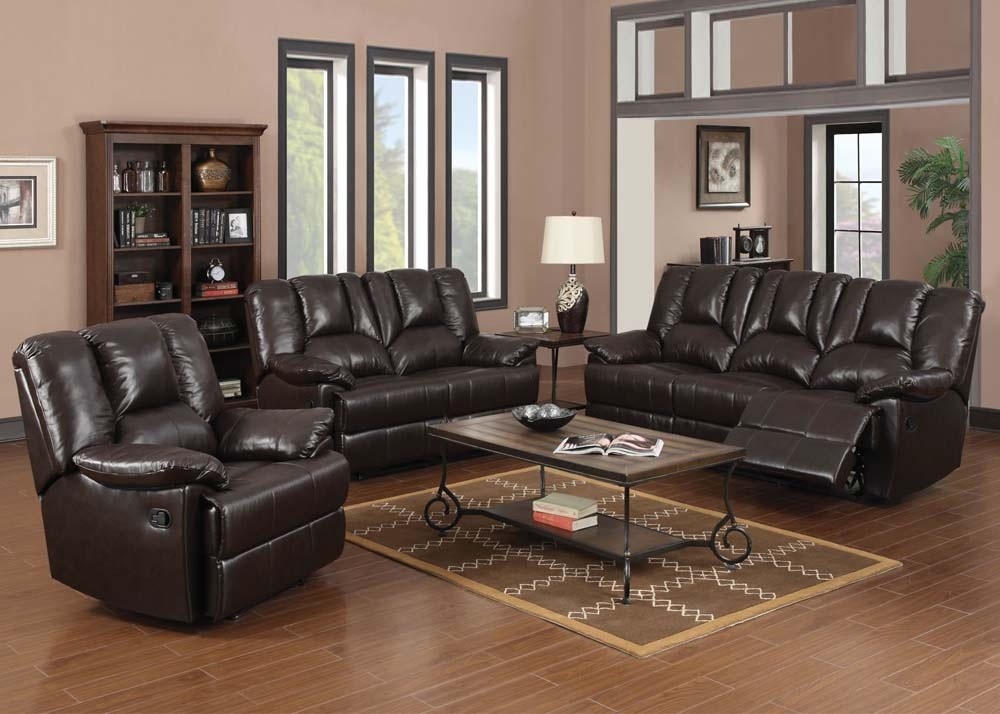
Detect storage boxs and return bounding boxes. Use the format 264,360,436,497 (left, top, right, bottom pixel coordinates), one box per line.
195,281,239,297
114,271,161,303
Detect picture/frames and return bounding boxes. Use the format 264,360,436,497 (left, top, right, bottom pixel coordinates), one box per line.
0,156,56,248
225,208,252,244
696,124,751,209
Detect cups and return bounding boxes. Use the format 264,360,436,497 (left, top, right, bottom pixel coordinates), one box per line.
155,281,173,300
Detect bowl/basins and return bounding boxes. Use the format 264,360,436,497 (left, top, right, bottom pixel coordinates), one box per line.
510,407,578,432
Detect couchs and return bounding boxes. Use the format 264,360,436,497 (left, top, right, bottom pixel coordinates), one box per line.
585,265,985,504
10,313,350,629
246,266,542,473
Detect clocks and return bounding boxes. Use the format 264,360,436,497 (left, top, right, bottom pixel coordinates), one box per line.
206,257,226,282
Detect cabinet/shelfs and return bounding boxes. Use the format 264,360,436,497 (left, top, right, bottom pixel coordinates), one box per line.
81,120,269,409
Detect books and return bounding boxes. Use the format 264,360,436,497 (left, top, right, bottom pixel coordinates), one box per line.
700,235,733,264
191,208,253,246
553,432,665,457
195,281,239,297
533,493,598,531
218,378,242,398
114,208,171,247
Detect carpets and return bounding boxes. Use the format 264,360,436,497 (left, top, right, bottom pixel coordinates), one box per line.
345,465,902,658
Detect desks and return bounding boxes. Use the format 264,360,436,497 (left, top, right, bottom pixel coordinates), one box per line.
496,327,609,411
425,414,752,603
667,259,793,272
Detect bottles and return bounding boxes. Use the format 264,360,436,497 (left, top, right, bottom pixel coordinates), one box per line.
141,160,155,192
193,149,231,192
134,161,143,192
122,161,138,193
112,164,123,193
157,161,172,192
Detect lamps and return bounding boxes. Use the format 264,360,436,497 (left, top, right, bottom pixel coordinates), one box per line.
540,211,606,333
